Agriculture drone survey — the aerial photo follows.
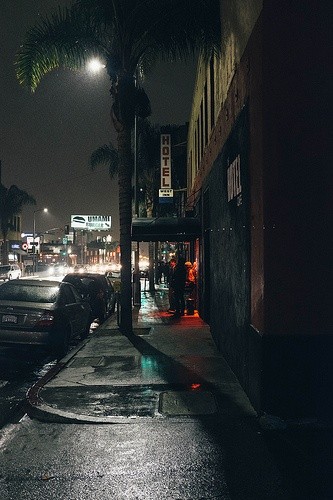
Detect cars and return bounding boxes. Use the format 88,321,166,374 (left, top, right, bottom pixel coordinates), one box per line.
0,276,94,353
48,263,150,321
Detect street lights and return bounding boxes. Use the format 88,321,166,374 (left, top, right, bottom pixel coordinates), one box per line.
33,208,48,276
86,56,141,307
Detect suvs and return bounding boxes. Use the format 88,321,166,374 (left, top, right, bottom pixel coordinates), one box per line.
0,265,22,281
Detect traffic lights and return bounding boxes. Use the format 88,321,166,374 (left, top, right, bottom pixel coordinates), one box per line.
62,252,66,256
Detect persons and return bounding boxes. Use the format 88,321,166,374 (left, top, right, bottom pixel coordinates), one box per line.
164,257,196,315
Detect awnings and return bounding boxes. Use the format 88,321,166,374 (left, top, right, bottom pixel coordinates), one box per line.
132,218,202,242
